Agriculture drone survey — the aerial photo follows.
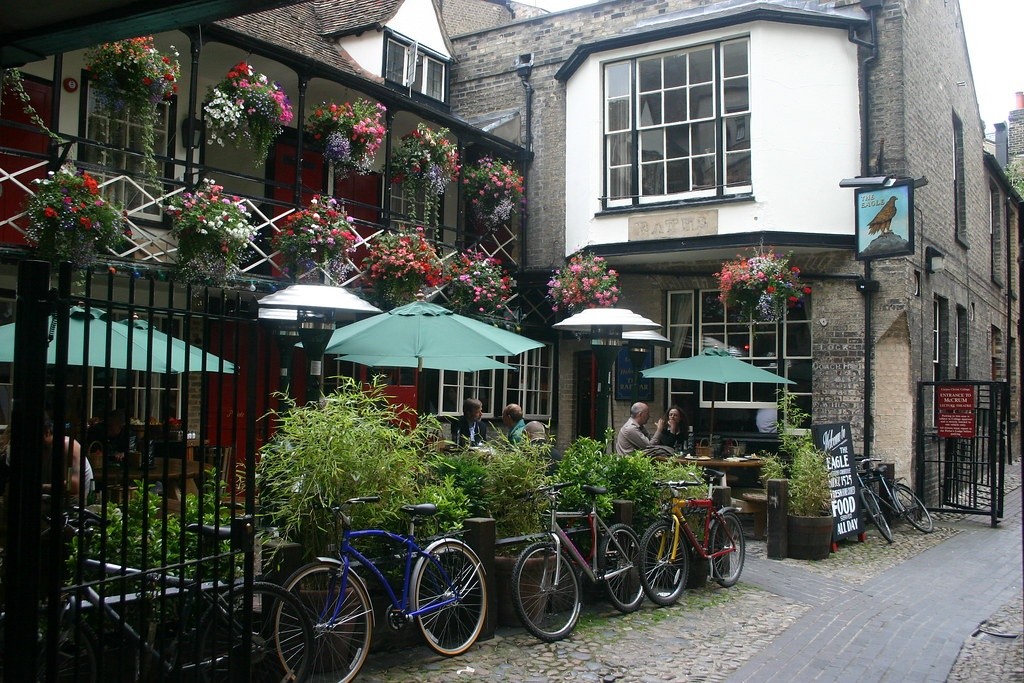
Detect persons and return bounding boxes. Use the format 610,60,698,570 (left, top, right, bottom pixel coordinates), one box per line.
86,408,129,456
616,402,665,458
451,397,487,447
502,402,526,444
41,420,96,508
660,405,688,450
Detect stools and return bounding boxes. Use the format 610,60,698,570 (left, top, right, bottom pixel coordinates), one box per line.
740,488,768,505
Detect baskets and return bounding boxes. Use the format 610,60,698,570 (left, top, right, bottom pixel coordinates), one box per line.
695,438,710,456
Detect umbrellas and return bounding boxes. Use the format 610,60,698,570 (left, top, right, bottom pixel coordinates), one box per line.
0,302,235,419
639,354,797,447
295,301,546,420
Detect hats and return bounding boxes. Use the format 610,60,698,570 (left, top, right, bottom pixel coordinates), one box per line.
522,421,546,444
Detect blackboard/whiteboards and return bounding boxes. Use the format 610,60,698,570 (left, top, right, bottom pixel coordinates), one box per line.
812,422,864,544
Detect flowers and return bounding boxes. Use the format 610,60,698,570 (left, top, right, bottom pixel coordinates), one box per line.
201,59,294,169
377,121,462,255
84,33,181,197
302,96,395,182
547,249,627,315
359,224,523,332
712,246,811,329
162,177,258,289
265,191,363,283
23,161,133,297
463,153,531,244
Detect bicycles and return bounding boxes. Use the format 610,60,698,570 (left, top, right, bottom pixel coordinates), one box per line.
636,473,746,606
854,457,933,543
270,497,488,683
508,482,645,643
1,503,315,683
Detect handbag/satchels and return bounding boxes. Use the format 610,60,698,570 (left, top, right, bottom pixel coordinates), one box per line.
721,438,741,456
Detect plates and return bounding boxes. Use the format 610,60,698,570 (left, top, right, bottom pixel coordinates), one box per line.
685,457,711,459
728,458,747,461
745,456,761,459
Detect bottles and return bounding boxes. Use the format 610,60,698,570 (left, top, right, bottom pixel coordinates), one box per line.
87,479,97,506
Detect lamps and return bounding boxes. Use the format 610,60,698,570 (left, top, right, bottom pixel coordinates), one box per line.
926,244,946,274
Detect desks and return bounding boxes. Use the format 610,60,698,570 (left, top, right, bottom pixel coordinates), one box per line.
153,438,209,461
656,453,776,540
93,456,213,503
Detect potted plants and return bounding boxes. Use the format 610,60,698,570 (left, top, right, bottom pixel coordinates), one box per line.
235,372,710,673
743,387,834,561
